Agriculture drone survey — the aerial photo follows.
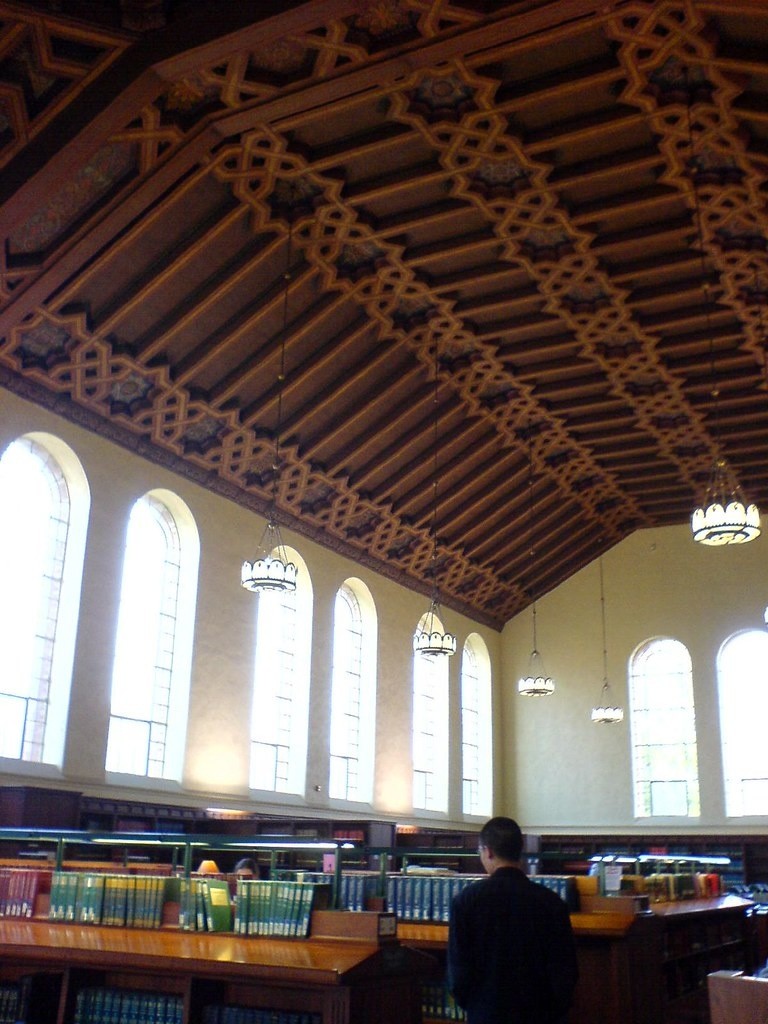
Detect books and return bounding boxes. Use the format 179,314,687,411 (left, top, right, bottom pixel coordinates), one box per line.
334,829,364,840
117,819,183,833
296,829,317,835
540,841,747,903
0,976,322,1024
422,981,467,1020
0,859,576,939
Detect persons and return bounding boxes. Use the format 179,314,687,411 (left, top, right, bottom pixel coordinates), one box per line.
447,817,579,1024
235,859,260,879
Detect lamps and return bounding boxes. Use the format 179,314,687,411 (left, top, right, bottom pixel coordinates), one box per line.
590,537,625,724
685,108,763,547
411,335,459,659
240,223,297,594
517,423,556,700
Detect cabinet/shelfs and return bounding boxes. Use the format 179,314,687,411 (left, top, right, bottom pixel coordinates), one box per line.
0,820,768,1024
